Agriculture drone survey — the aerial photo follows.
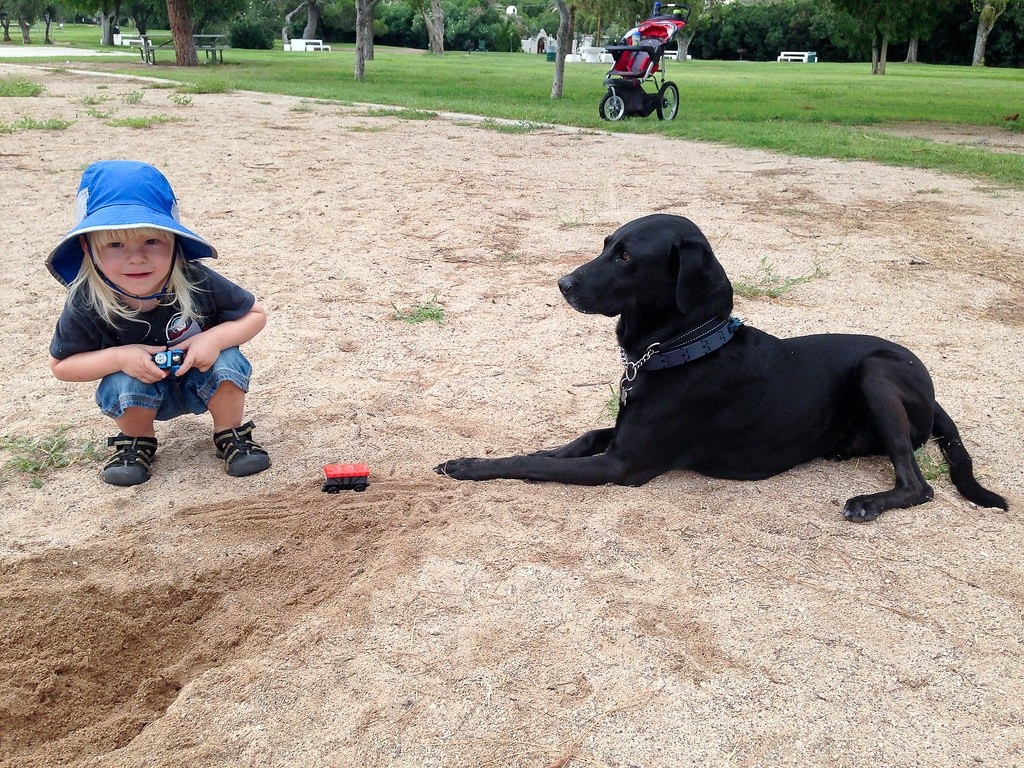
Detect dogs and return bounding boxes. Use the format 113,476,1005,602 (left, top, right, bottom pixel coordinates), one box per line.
429,213,1009,525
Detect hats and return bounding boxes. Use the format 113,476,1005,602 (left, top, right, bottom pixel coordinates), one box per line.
45,160,218,290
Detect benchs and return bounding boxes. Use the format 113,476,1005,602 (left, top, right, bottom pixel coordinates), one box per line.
283,44,291,51
305,45,331,51
143,45,231,51
815,57,818,62
121,40,152,45
132,45,201,49
660,55,676,60
777,56,805,63
687,55,691,60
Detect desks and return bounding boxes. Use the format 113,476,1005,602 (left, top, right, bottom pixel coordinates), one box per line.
781,52,816,62
113,34,150,46
138,35,226,65
291,39,322,51
664,50,678,56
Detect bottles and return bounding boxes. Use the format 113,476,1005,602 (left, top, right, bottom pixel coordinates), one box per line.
632,31,640,46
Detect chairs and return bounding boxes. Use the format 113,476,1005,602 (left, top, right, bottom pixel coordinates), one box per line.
57,23,64,31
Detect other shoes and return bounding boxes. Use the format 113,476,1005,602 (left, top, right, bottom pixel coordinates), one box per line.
104,432,157,486
213,420,272,476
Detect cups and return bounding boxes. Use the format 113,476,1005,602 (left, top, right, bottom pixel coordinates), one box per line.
654,1,660,14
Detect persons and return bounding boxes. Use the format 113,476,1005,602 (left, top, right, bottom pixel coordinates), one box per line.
46,158,272,487
